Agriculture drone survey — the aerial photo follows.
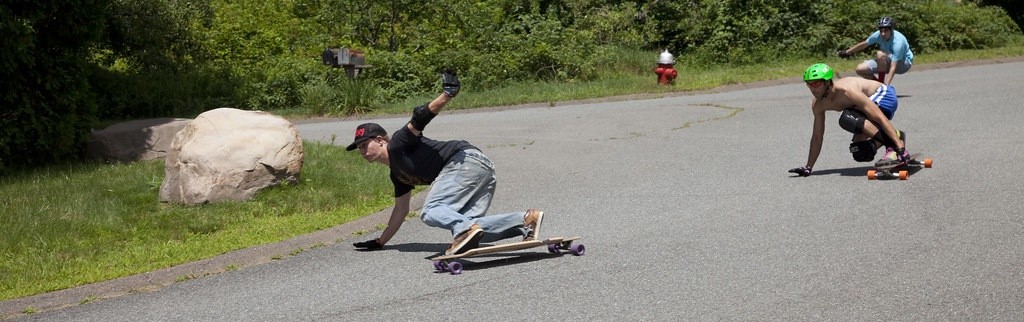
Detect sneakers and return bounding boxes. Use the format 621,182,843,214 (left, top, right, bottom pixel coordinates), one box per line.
875,146,901,166
522,208,545,242
894,129,906,148
444,223,484,255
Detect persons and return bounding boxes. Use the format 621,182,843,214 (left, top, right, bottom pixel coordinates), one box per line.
788,63,910,177
346,66,543,256
839,16,915,85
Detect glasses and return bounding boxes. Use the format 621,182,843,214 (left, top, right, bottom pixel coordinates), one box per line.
807,81,825,88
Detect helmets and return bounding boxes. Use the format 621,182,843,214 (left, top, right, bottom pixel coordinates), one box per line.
877,16,897,30
803,63,834,81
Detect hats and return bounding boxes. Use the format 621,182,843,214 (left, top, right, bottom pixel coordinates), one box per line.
346,122,388,152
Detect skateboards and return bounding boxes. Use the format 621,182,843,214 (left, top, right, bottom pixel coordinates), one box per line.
866,150,934,177
429,235,589,275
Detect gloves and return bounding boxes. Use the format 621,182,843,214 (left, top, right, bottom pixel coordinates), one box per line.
837,50,851,59
896,147,911,163
440,70,460,97
353,238,382,250
788,165,811,177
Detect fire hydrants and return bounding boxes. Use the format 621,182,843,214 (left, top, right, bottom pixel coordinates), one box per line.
653,48,678,85
